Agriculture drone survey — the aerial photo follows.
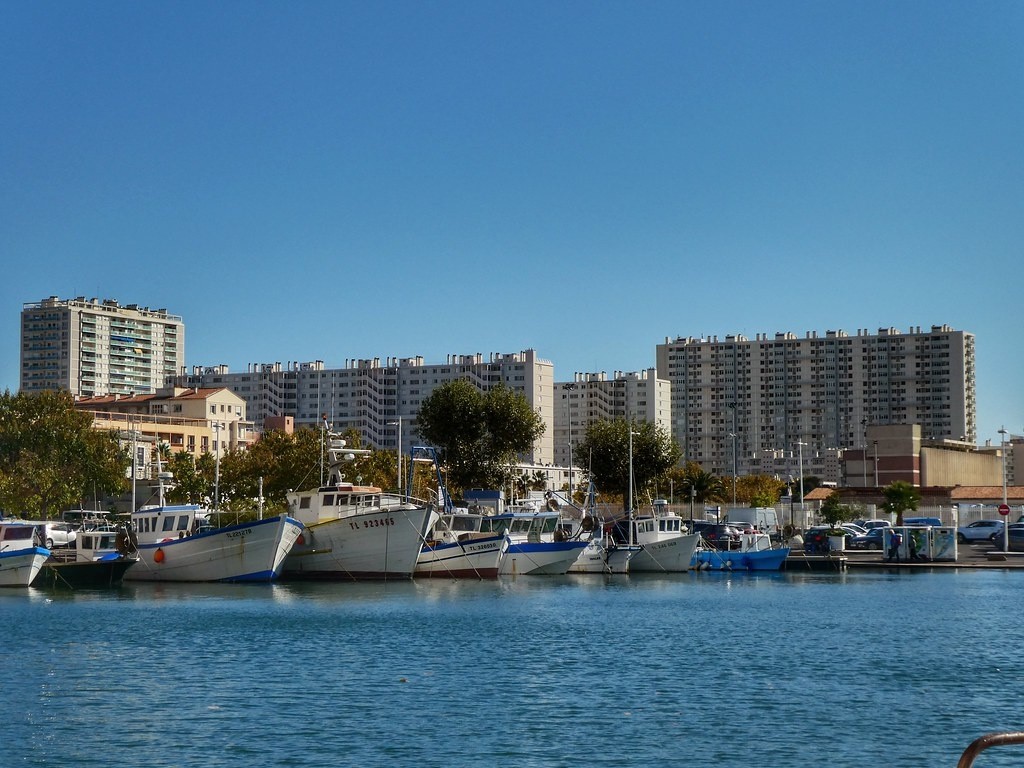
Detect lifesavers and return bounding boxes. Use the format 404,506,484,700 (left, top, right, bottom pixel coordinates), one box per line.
555,530,568,542
115,530,138,555
582,515,600,532
783,525,794,538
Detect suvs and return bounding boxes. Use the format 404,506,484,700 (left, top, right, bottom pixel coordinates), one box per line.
802,517,942,554
958,519,1004,546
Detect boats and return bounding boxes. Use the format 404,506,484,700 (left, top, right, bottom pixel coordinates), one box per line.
0,412,791,589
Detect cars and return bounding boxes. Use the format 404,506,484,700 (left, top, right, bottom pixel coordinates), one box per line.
994,515,1024,552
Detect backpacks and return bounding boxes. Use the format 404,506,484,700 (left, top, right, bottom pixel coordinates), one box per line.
896,534,902,545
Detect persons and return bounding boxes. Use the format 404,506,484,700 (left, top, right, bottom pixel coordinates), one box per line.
909,535,918,559
889,529,900,560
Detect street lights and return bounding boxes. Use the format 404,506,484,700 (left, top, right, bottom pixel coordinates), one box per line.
628,426,640,545
213,417,225,509
792,437,808,509
130,429,140,514
727,401,739,508
563,382,576,497
386,416,403,494
998,425,1008,553
860,417,868,490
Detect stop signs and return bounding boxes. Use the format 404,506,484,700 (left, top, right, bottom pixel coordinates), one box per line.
998,503,1011,516
